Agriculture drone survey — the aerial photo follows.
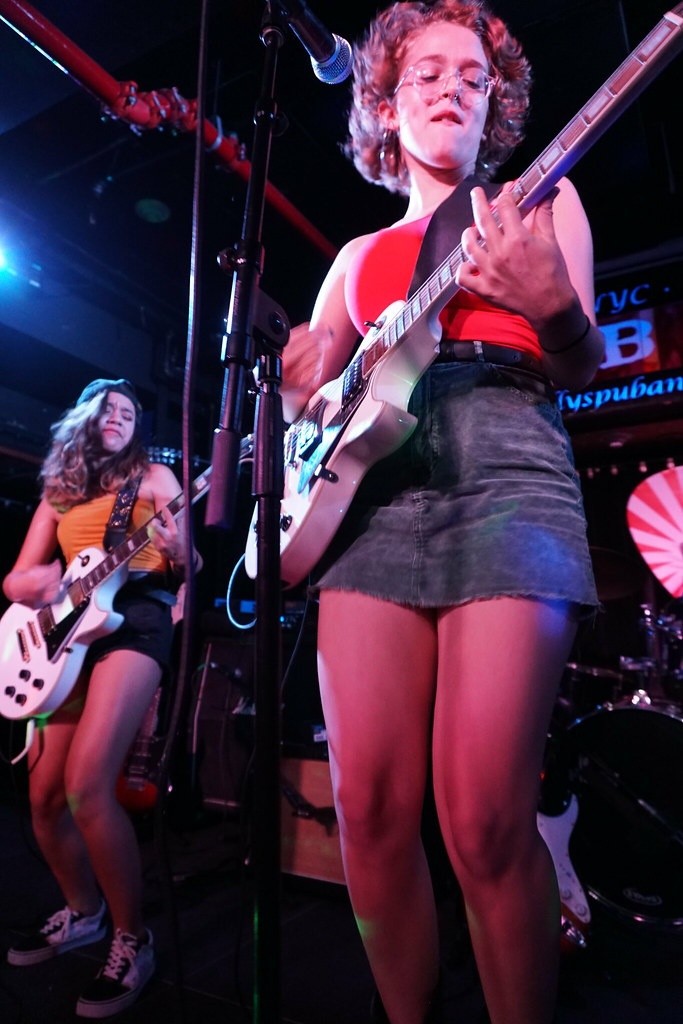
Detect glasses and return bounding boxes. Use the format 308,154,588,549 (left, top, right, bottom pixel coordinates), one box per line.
391,65,497,106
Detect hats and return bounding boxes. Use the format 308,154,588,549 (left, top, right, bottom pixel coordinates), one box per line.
76,379,143,425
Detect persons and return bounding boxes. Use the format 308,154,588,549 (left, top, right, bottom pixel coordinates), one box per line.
2,379,204,1018
260,0,599,1024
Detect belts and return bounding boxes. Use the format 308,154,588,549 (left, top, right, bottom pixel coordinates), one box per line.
434,334,551,380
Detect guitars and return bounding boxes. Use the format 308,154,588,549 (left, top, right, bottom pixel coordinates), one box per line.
243,0,683,588
0,432,258,720
115,686,161,812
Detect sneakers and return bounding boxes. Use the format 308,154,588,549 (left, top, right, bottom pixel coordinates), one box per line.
78,925,156,1018
8,897,107,965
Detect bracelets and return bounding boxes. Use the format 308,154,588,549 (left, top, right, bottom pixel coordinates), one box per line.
538,314,591,355
192,553,198,573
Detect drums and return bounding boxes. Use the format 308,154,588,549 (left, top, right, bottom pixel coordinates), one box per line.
554,597,682,719
535,693,683,931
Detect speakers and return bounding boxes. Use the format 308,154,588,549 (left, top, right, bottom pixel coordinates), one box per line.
271,739,350,892
201,637,330,811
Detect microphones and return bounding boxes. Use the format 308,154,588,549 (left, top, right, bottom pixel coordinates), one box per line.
277,0,354,84
291,806,338,824
